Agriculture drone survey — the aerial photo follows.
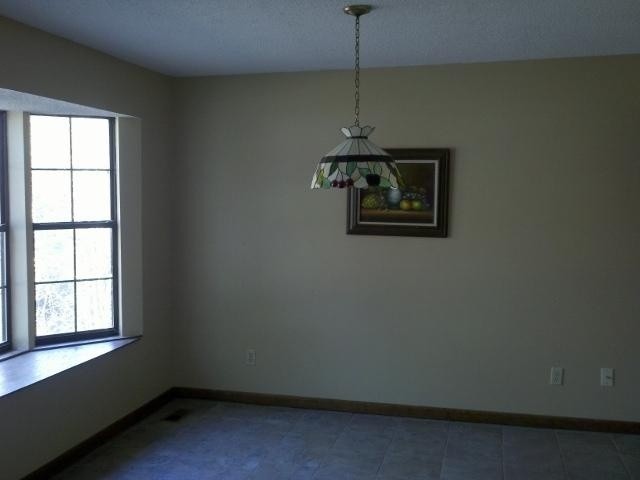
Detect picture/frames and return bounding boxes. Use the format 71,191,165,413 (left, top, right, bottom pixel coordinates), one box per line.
345,147,452,238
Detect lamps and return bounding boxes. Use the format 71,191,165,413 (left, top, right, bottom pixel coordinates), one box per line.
310,4,406,191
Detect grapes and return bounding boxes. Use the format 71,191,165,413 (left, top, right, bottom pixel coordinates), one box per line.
402,191,431,208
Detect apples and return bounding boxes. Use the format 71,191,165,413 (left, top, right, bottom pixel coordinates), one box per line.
400,199,423,211
